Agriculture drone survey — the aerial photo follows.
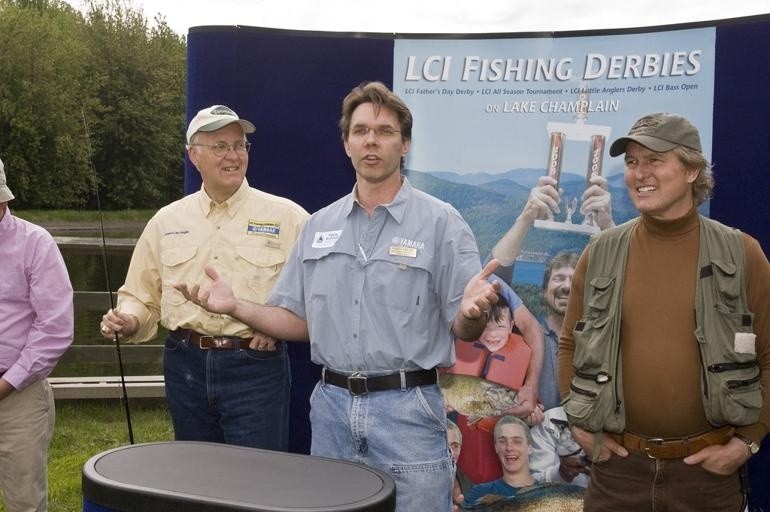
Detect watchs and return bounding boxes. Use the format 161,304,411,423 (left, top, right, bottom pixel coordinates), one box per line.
733,432,760,454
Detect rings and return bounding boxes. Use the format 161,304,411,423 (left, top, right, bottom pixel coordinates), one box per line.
101,327,108,334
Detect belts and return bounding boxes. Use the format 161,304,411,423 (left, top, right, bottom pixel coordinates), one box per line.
611,426,735,459
324,369,437,396
176,328,253,349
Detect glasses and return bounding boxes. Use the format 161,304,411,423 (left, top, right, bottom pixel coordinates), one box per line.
353,127,402,138
191,141,252,157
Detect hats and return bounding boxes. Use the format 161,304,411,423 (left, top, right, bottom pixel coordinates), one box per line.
0,158,15,203
186,105,256,144
610,112,700,157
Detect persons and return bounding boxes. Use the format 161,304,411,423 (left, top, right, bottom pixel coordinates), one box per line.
171,79,507,511
463,414,540,508
0,159,77,511
481,269,545,420
481,174,617,410
527,406,589,488
95,97,316,453
556,111,770,512
438,292,531,391
445,416,464,512
